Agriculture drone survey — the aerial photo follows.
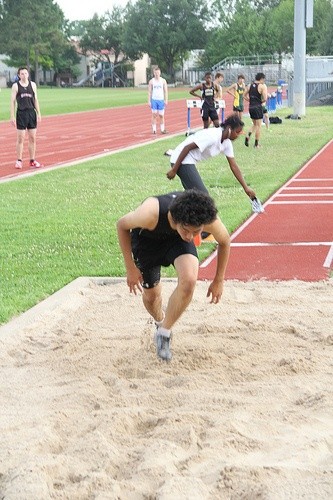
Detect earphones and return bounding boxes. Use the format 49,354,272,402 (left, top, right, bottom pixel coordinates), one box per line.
228,128,231,134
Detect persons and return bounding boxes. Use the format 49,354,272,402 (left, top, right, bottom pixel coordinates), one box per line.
243,73,267,148
148,68,168,134
261,83,269,131
189,72,220,128
10,68,42,169
166,114,256,239
116,187,230,360
209,73,224,125
226,75,245,120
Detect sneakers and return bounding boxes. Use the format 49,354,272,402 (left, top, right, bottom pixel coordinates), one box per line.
16,160,22,168
250,196,264,213
157,335,171,360
29,161,40,168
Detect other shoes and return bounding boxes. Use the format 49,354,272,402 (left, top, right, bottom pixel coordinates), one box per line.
245,137,248,146
161,130,167,134
201,233,215,242
255,145,260,149
